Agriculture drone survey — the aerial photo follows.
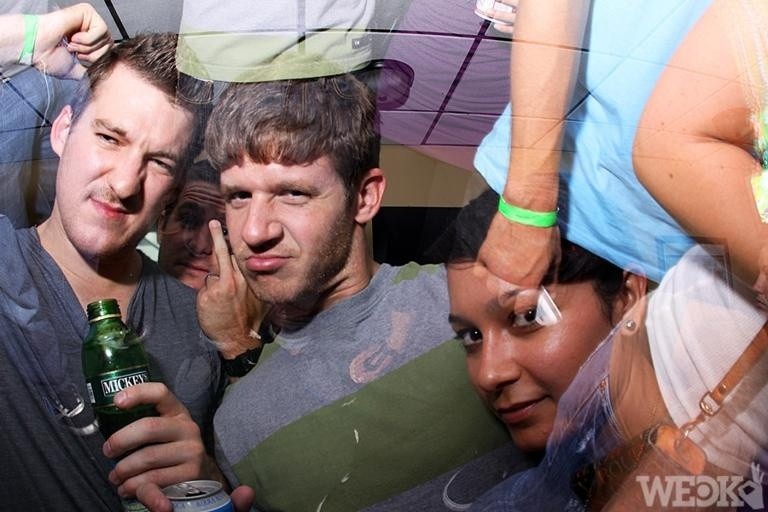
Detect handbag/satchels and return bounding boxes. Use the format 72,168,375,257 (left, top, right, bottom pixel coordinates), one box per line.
569,422,768,512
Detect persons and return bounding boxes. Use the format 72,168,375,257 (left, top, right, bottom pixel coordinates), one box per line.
0,1,768,512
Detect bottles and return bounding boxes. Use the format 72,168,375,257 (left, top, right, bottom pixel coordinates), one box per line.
84,295,158,442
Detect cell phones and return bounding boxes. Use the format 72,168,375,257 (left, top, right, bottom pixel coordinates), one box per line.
533,283,563,328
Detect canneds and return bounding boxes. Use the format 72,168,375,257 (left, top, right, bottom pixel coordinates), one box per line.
161,480,236,512
474,0,517,26
